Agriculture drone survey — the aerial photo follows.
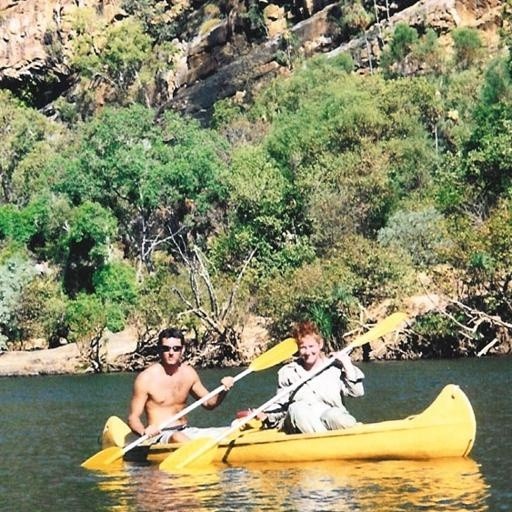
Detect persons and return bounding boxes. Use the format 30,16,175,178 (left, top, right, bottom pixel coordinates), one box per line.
246,319,367,436
126,326,237,447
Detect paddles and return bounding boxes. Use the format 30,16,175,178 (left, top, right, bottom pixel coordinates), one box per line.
157,311,408,470
79,337,298,469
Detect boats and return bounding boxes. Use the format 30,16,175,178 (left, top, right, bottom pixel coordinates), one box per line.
98,381,480,468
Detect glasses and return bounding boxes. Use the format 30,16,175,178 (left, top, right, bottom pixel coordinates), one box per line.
160,344,182,353
